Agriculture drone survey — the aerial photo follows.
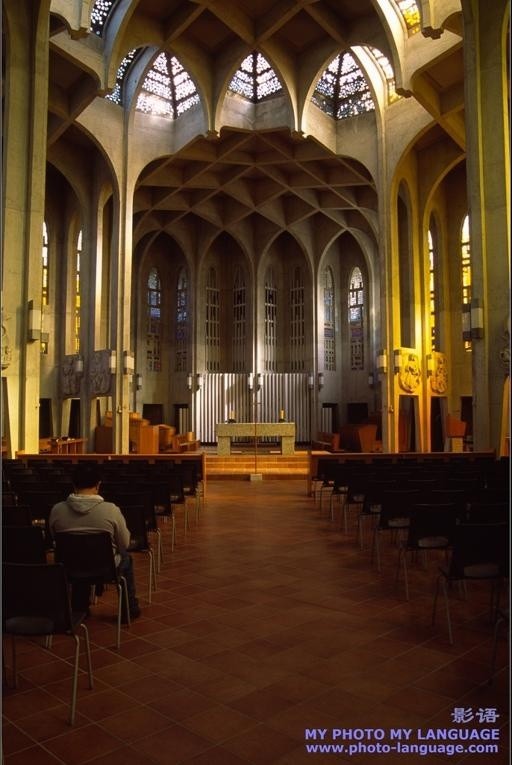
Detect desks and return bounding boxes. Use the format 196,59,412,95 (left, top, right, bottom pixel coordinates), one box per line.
215,423,296,455
308,450,496,497
48,438,86,454
16,449,206,497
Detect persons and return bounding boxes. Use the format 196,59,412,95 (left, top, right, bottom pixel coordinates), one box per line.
50,462,139,623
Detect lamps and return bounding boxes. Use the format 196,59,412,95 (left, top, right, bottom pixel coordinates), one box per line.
27,298,484,391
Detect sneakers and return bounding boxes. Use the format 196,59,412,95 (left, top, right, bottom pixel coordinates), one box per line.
117,598,141,625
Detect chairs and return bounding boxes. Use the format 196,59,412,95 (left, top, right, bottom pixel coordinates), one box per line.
311,458,510,686
1,459,204,727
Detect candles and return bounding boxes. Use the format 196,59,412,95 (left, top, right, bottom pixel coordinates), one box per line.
229,411,235,419
279,410,284,419
